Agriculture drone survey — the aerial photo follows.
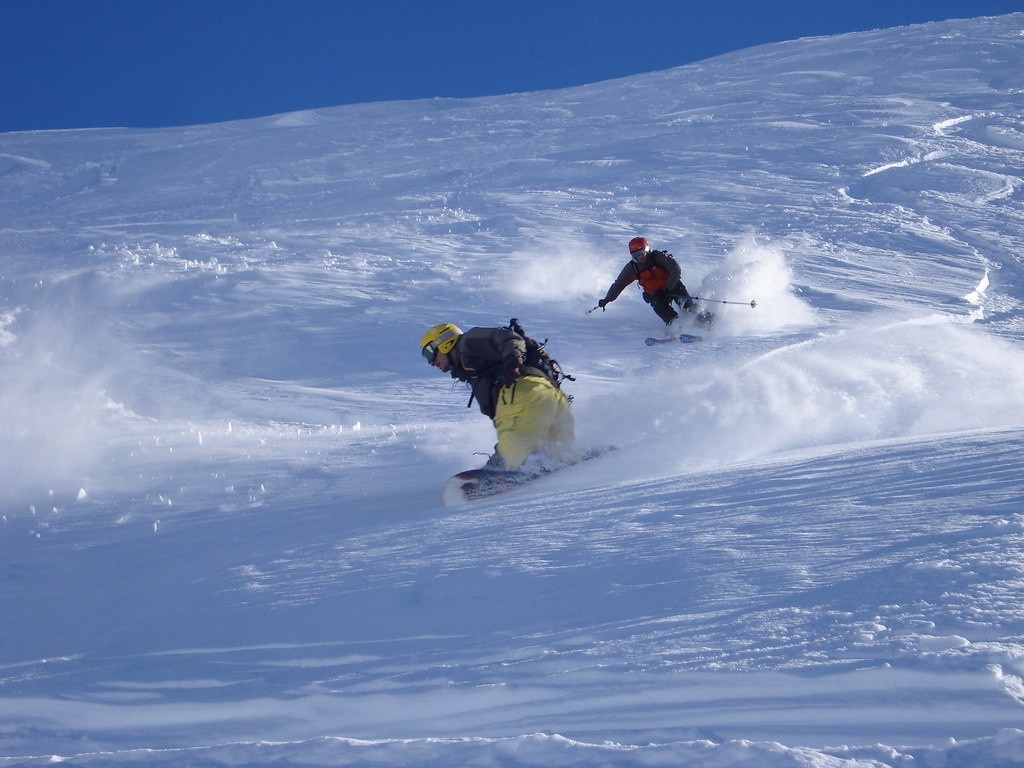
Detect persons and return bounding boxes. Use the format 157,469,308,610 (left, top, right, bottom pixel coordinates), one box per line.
420,318,575,471
598,237,698,327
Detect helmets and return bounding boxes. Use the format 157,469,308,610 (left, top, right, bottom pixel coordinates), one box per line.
629,237,650,255
420,323,463,354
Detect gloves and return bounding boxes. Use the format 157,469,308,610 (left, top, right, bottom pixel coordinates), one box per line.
654,288,668,302
502,351,522,388
599,299,609,307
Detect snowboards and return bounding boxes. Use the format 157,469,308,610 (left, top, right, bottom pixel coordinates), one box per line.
443,441,619,509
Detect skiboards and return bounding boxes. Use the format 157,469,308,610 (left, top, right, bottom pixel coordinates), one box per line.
644,332,704,346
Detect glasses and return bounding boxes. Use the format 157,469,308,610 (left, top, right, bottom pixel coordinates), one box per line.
630,248,647,258
422,343,437,364
